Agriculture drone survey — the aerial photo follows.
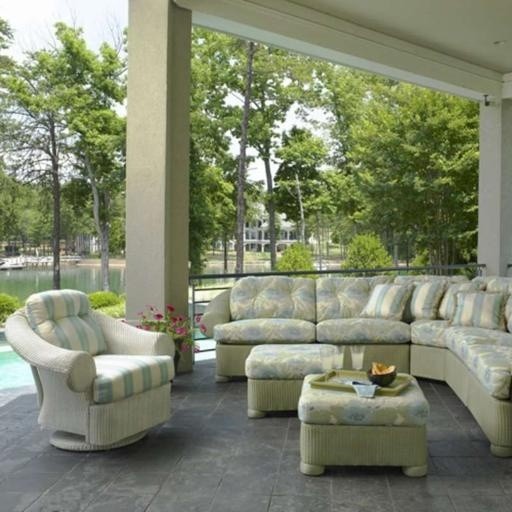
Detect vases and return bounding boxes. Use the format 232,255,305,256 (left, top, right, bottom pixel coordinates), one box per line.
173,350,181,375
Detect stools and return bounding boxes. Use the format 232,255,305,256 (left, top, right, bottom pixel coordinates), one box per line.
244,340,342,419
294,368,429,480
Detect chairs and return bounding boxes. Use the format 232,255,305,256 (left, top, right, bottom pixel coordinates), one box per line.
4,286,178,455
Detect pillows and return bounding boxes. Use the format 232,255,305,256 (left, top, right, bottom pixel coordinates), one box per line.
359,283,414,320
438,279,486,321
448,289,506,331
402,281,444,321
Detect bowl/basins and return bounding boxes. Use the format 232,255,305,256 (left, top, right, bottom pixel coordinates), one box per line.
367,366,396,386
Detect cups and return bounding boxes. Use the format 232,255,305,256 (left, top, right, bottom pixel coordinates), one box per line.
333,345,345,370
349,344,365,370
319,346,335,373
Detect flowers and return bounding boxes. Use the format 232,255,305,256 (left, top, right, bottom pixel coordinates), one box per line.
122,303,208,353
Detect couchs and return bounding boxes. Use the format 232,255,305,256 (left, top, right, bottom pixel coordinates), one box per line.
198,274,512,459
